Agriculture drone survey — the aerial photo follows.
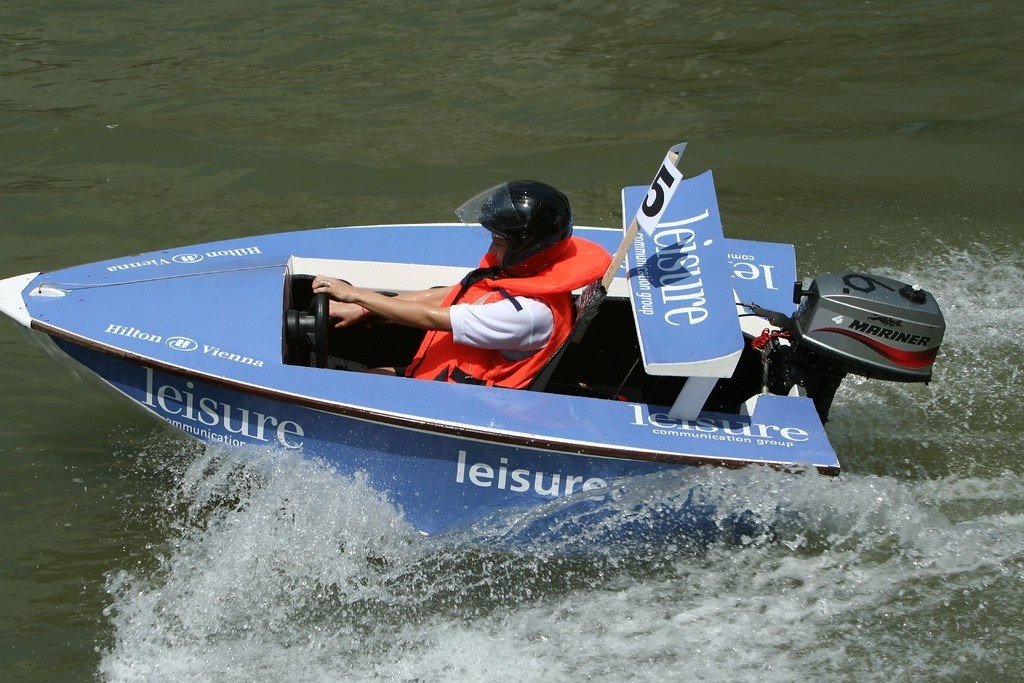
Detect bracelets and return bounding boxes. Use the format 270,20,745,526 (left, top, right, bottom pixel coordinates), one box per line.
362,307,371,316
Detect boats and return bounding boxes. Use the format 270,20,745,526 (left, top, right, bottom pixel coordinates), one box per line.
0,221,943,561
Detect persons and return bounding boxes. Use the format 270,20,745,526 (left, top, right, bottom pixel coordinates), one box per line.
311,180,575,391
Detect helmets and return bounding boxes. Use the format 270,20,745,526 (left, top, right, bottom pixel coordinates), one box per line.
478,180,573,267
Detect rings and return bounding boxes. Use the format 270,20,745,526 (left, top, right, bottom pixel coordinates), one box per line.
321,281,330,286
